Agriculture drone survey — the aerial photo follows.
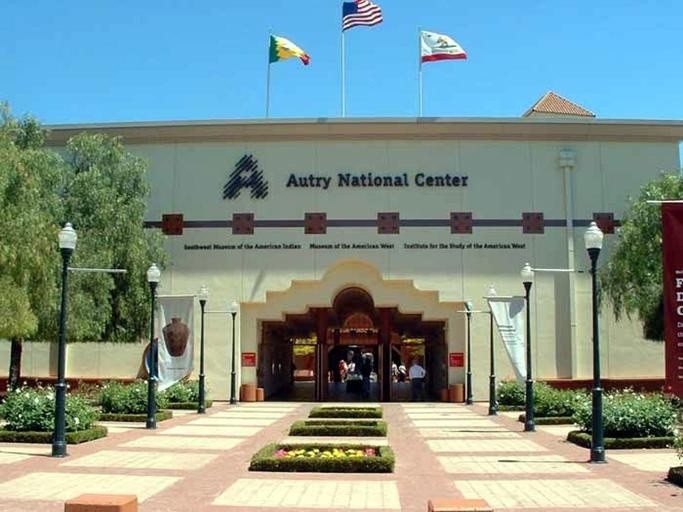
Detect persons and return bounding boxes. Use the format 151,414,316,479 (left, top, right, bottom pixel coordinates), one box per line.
338,350,406,391
406,357,428,401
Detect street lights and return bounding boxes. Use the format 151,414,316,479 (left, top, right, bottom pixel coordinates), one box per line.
229,297,237,404
464,299,472,405
520,262,534,430
50,221,76,457
197,284,208,414
486,285,497,415
583,221,607,463
146,263,160,430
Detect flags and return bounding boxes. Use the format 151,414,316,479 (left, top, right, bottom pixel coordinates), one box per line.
269,35,310,66
486,298,527,385
342,0,383,31
154,294,193,393
660,201,683,399
421,30,468,63
